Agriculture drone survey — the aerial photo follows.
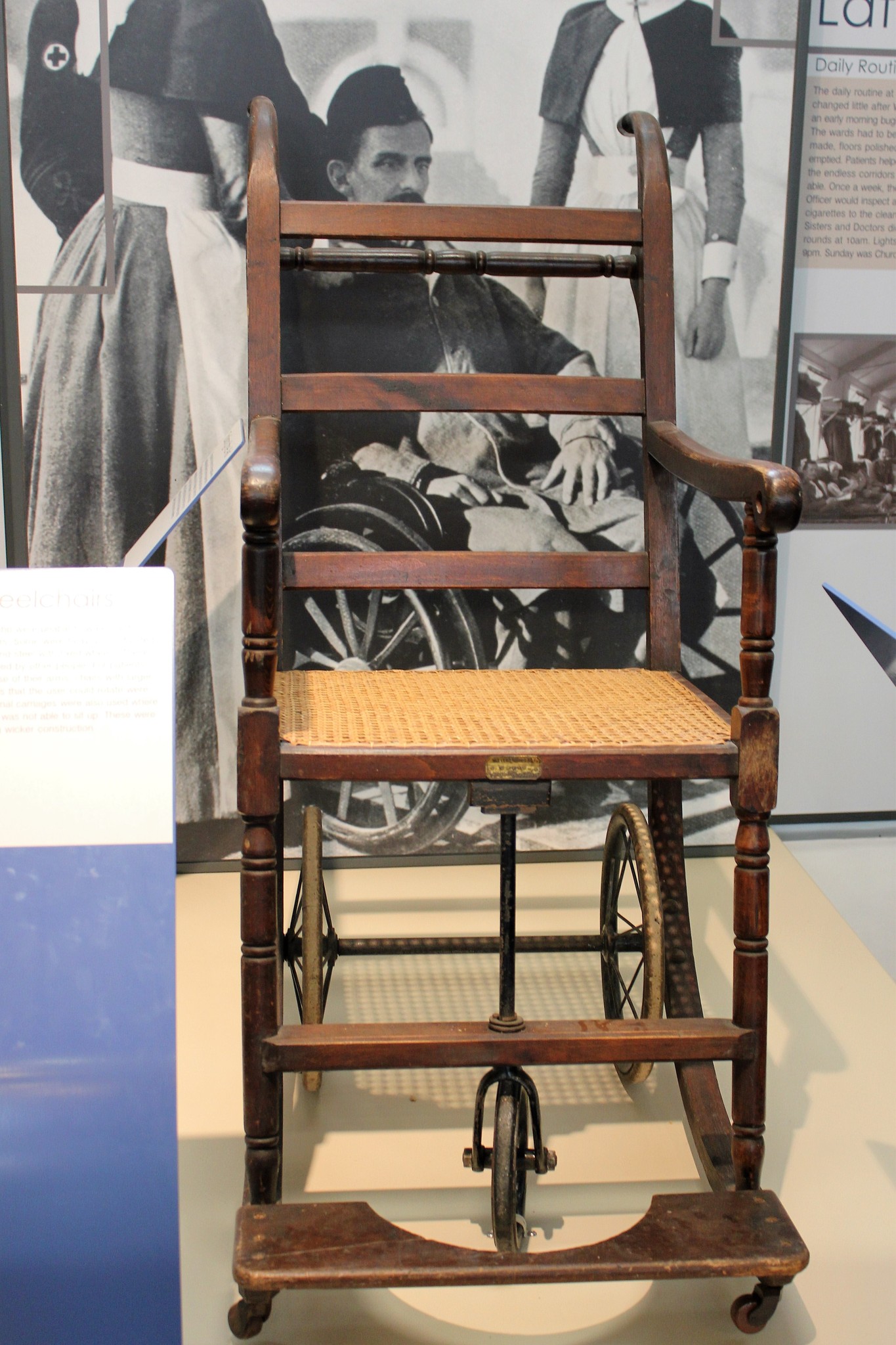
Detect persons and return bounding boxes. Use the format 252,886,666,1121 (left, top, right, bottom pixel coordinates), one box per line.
17,1,754,881
800,444,896,517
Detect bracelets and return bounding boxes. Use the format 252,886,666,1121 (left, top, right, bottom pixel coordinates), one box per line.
702,241,737,286
705,235,740,244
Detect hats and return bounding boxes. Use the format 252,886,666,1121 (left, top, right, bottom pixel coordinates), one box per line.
327,64,421,160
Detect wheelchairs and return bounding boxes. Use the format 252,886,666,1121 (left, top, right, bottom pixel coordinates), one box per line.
281,432,756,855
